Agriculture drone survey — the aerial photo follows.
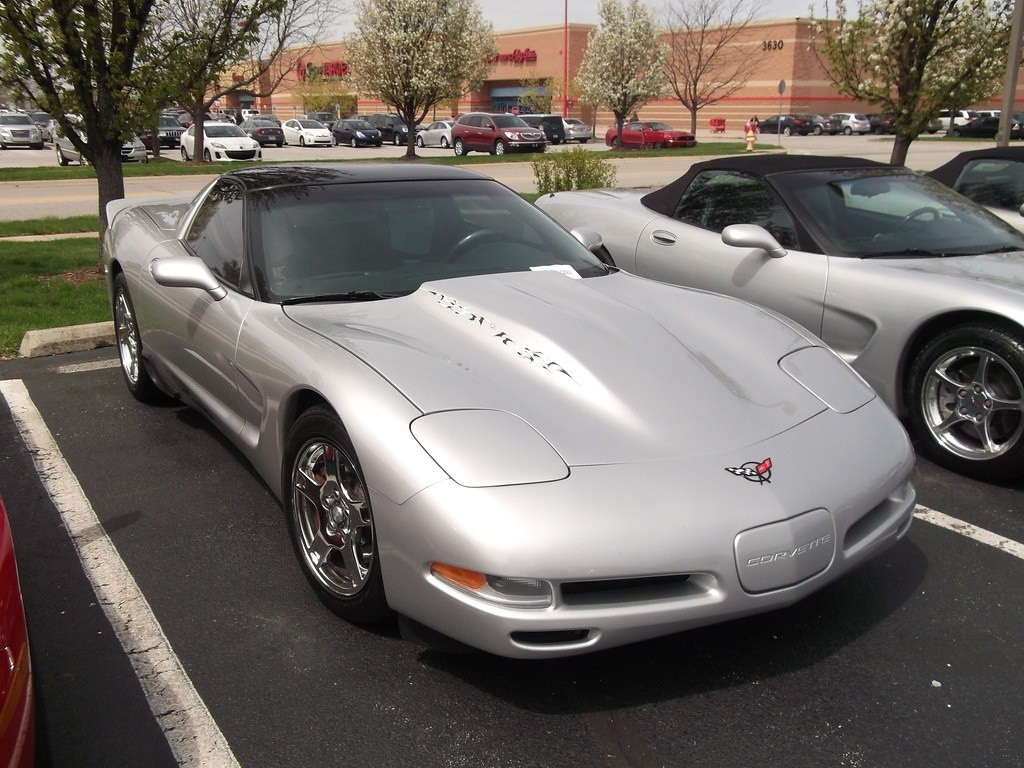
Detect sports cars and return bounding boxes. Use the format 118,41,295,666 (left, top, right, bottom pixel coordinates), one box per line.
522,154,1024,485
102,158,921,663
840,145,1024,237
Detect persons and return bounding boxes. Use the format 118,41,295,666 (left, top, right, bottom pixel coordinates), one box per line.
751,115,760,128
629,114,639,122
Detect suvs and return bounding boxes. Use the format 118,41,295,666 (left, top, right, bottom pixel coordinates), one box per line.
367,112,428,146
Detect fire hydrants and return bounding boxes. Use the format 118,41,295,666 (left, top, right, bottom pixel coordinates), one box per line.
744,126,757,151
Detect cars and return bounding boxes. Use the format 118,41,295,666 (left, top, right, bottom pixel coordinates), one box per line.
450,111,549,157
0,108,54,150
754,107,1024,137
505,112,592,146
179,120,263,163
604,119,697,151
133,106,285,148
52,113,148,167
298,111,372,132
331,118,384,148
281,118,337,147
945,116,1024,140
415,121,456,149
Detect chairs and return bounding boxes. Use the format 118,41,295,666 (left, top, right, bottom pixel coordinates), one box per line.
376,199,465,257
267,207,349,265
734,184,847,235
